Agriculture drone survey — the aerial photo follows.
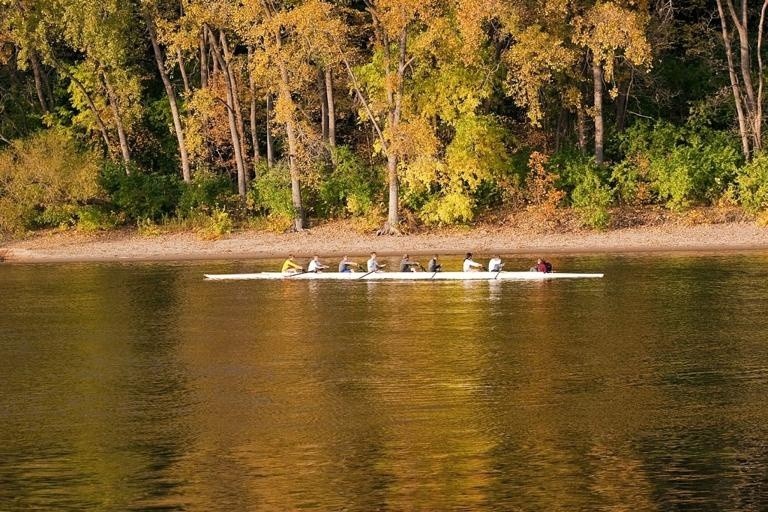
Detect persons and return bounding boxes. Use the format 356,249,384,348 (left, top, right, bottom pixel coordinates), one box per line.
338,254,359,273
366,251,387,273
281,254,303,276
427,254,445,272
529,256,548,275
399,253,421,272
462,252,485,272
307,255,329,273
488,253,505,273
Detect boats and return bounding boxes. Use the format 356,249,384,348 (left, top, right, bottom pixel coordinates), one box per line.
203,272,605,280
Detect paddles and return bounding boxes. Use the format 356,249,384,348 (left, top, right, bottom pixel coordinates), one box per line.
352,266,382,280
283,268,323,277
